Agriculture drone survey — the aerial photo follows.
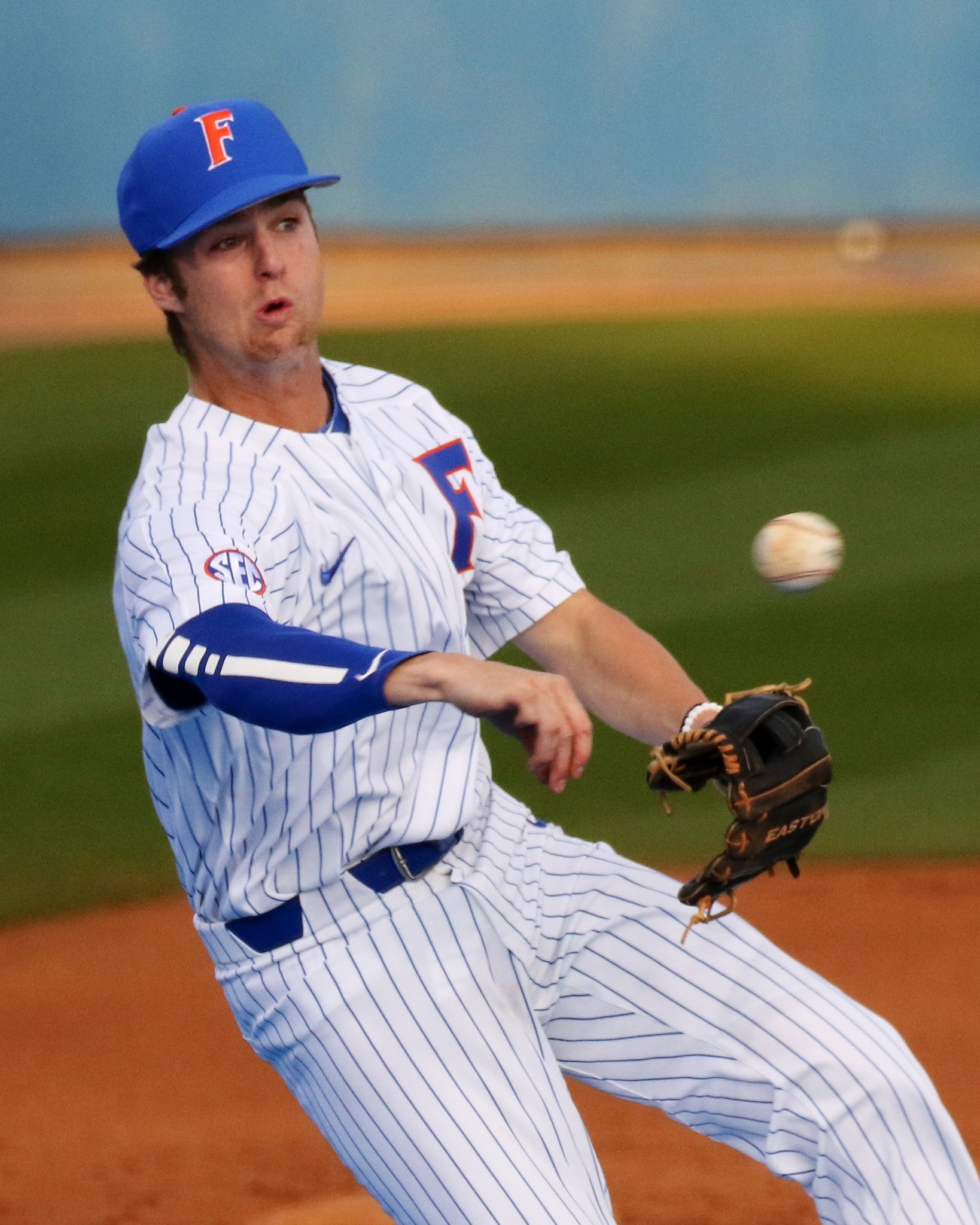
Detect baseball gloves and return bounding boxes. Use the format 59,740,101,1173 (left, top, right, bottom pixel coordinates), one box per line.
647,677,842,911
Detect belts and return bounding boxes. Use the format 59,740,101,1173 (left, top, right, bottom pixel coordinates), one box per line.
226,828,463,952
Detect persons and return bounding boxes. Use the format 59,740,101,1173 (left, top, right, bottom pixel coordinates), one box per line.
110,96,980,1225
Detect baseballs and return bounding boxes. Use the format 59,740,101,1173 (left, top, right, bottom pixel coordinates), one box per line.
751,510,845,599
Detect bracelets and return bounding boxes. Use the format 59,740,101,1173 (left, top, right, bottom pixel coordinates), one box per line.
680,703,702,732
682,701,723,733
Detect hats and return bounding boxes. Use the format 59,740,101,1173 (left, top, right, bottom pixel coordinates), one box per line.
118,98,340,254
